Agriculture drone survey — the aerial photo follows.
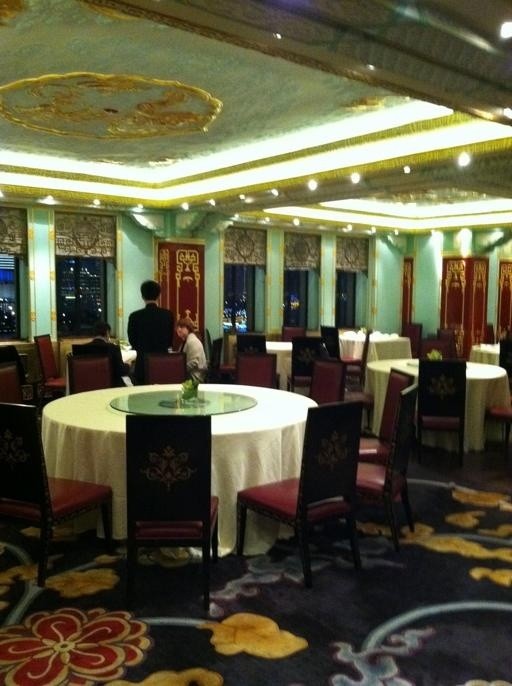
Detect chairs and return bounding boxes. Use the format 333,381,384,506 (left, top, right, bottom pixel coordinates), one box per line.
124,413,221,604
234,400,364,585
0,404,113,587
412,357,468,465
0,324,417,553
487,406,511,454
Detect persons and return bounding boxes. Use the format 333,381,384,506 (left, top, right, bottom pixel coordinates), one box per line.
176,318,208,383
127,279,174,386
83,322,132,387
